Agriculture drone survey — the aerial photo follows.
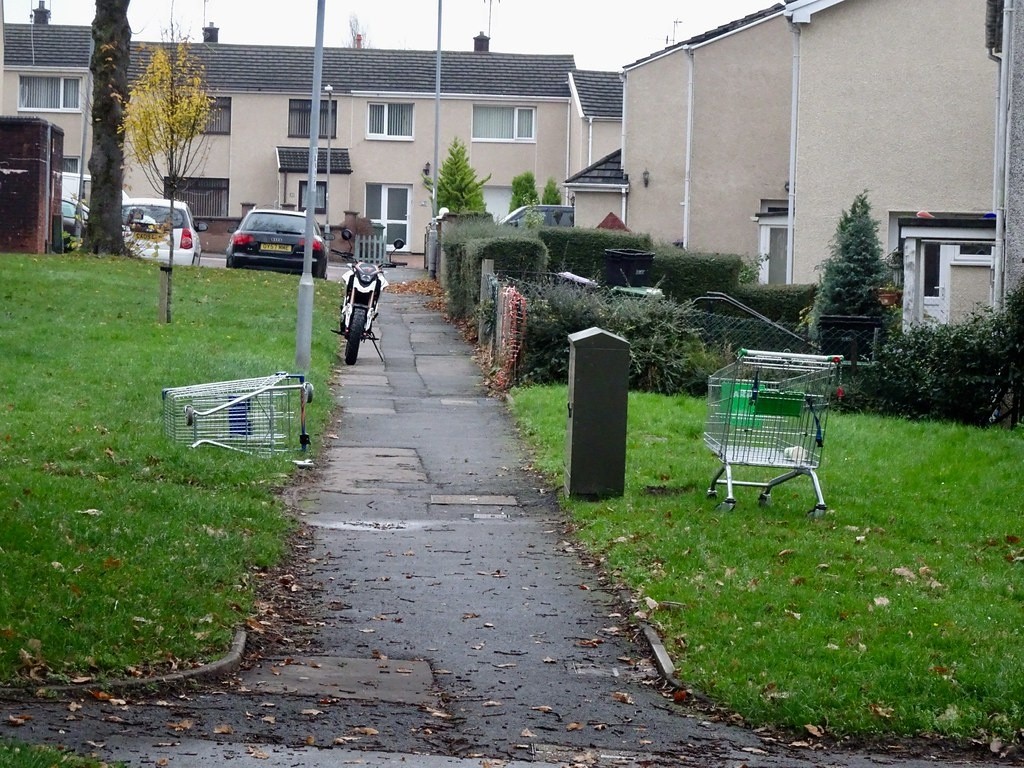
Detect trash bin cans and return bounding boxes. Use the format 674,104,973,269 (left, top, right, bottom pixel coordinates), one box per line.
603,247,655,286
361,223,386,263
614,284,663,300
818,315,882,362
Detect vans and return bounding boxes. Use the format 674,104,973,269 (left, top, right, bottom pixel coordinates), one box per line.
62,172,130,209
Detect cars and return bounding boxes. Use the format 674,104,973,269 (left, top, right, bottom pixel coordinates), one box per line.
493,204,575,226
121,197,208,267
61,195,132,243
225,204,336,278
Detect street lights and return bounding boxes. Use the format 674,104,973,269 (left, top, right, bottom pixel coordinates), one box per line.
323,84,334,255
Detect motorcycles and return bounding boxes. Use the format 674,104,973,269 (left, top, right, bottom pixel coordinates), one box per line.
330,227,408,366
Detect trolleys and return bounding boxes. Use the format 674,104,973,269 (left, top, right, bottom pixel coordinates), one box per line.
160,371,315,461
701,348,845,518
